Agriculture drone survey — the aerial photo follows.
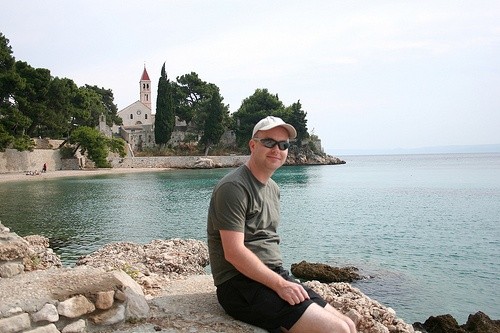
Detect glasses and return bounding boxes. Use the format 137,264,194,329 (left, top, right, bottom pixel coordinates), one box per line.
254,137,291,151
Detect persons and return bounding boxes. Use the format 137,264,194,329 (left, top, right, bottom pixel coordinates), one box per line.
206,116,357,333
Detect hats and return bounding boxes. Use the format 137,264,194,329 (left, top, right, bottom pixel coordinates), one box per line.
251,116,297,139
